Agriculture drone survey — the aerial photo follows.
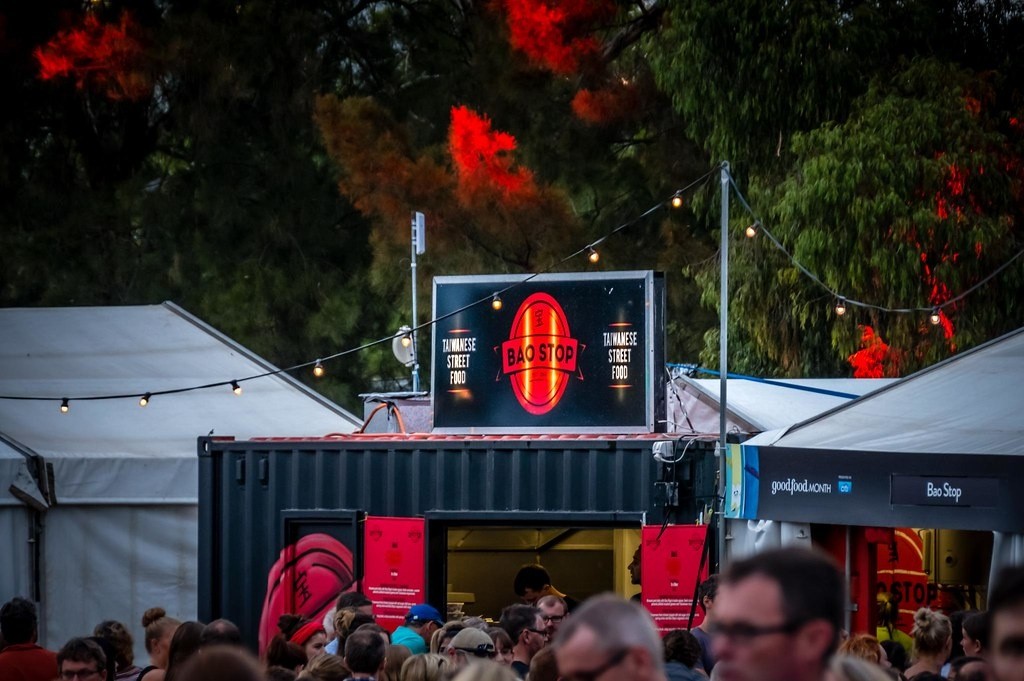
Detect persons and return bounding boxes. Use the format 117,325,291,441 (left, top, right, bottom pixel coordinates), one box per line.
324,591,391,681
904,607,953,681
500,564,584,681
0,596,61,681
390,604,514,681
953,567,1024,681
56,638,108,681
841,631,912,681
628,543,642,605
662,576,722,681
163,619,262,681
711,548,895,681
93,620,144,681
136,607,182,681
553,595,668,681
262,615,352,681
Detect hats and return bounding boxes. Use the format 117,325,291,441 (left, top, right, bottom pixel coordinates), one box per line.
405,603,445,626
451,627,496,664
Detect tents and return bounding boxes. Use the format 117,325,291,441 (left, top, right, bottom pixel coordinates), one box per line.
725,326,1024,636
0,300,365,670
667,369,903,435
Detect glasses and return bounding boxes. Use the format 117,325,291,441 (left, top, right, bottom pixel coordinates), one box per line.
448,630,458,637
543,613,566,624
559,649,630,681
519,627,547,637
455,645,498,660
62,668,98,678
712,621,788,644
